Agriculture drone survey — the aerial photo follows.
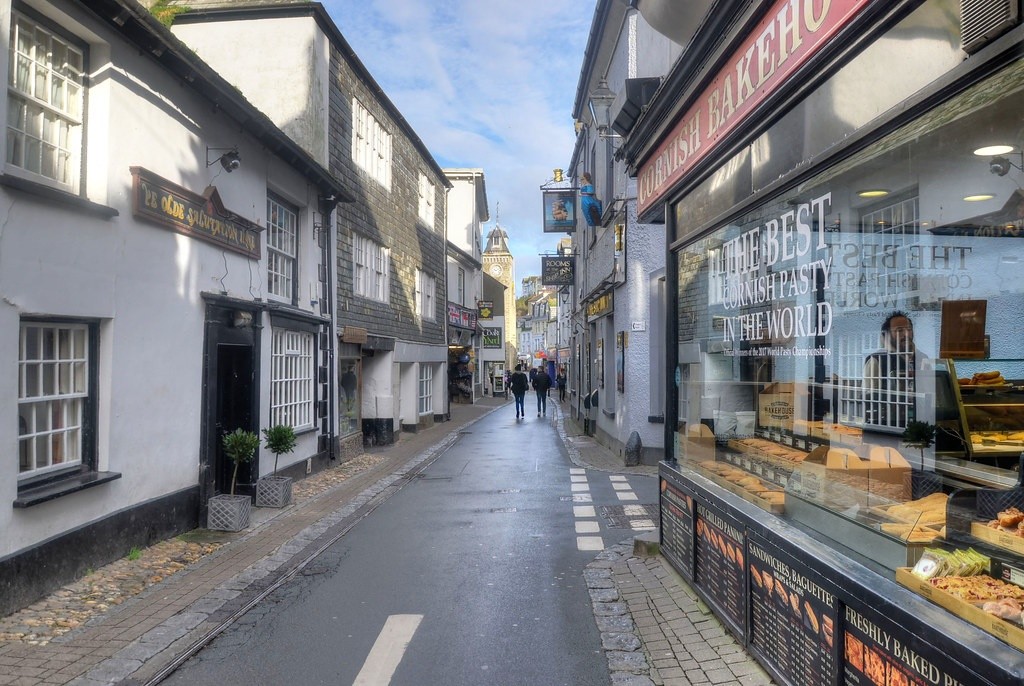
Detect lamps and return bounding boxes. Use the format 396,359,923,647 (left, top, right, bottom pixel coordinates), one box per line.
587,75,622,150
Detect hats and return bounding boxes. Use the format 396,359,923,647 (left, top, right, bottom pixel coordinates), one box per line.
516,364,521,370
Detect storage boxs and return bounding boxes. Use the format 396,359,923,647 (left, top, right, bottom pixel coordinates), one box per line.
800,442,912,510
758,382,812,431
673,423,716,462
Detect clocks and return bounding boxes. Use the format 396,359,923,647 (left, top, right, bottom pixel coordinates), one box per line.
489,263,503,278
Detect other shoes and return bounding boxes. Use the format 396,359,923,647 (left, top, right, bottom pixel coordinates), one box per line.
562,399,565,402
538,412,545,417
521,416,524,418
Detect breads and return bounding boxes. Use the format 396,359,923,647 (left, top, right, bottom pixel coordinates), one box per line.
986,507,1024,539
970,431,1024,444
879,493,951,542
958,371,1004,386
738,439,808,463
795,420,862,435
697,461,784,503
928,575,1024,625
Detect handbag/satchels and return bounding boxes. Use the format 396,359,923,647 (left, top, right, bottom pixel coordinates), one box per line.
523,374,529,390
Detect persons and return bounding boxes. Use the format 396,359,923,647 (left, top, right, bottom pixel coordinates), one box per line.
556,368,567,403
506,364,529,420
531,365,551,418
861,310,932,400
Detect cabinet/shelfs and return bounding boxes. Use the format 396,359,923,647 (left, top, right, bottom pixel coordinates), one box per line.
919,357,1024,471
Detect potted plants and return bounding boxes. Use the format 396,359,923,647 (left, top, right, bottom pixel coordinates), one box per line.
207,427,263,532
255,424,300,508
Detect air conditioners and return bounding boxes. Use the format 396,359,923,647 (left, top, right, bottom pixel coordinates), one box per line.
959,0,1024,56
